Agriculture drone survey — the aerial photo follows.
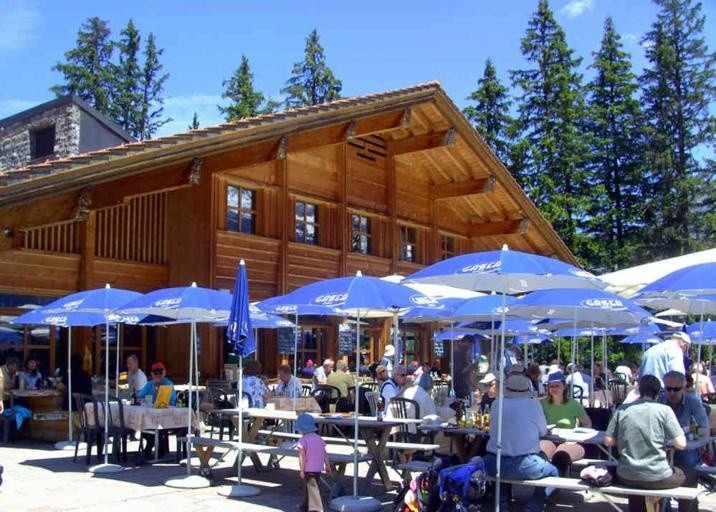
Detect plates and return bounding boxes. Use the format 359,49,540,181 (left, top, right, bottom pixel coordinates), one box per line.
318,413,350,419
439,422,458,429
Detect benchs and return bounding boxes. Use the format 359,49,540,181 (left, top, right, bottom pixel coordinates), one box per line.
186,432,707,512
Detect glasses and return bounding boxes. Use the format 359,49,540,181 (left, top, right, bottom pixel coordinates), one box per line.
665,387,682,391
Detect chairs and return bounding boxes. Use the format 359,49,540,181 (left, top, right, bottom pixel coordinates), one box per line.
72,392,142,466
205,372,716,461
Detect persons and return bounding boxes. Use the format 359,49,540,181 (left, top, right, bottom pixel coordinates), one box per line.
0,332,716,511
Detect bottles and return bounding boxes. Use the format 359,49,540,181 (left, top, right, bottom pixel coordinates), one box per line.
375,397,385,423
181,395,185,406
474,404,483,431
689,411,698,440
129,387,136,406
483,404,491,432
271,386,275,396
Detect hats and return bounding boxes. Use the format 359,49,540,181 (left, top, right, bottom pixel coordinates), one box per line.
151,362,164,371
383,344,395,357
671,331,691,347
293,414,318,433
479,370,507,384
545,371,565,384
502,374,532,397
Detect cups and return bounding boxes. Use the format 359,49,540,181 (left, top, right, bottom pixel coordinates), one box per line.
121,399,127,405
329,404,336,414
688,433,693,441
463,412,474,431
